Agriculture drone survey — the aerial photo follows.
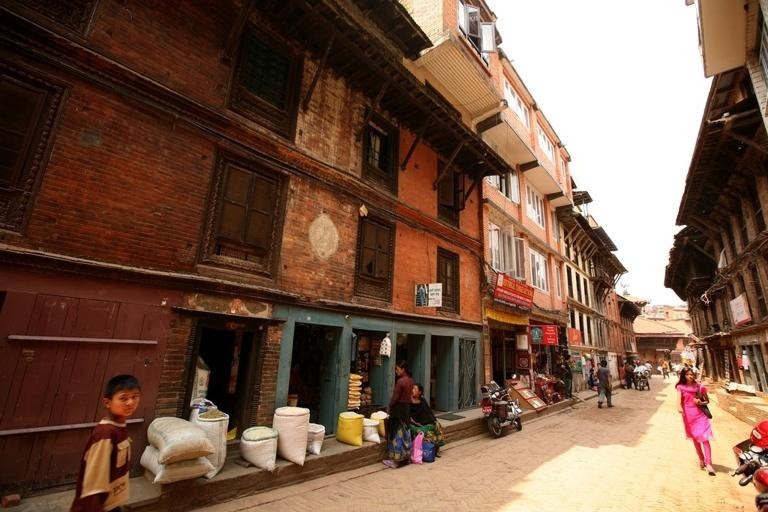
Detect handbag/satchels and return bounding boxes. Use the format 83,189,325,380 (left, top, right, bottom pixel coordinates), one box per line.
422,442,435,462
700,398,712,418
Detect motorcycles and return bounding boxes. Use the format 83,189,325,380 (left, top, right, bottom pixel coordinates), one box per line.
734,420,768,511
481,373,522,437
634,371,649,390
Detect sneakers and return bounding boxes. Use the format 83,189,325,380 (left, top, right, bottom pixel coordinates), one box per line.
699,461,716,476
382,459,399,469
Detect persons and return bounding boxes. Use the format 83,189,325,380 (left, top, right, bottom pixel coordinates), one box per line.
587,357,699,408
381,359,414,470
673,367,716,477
559,354,575,400
67,373,142,511
408,385,447,459
